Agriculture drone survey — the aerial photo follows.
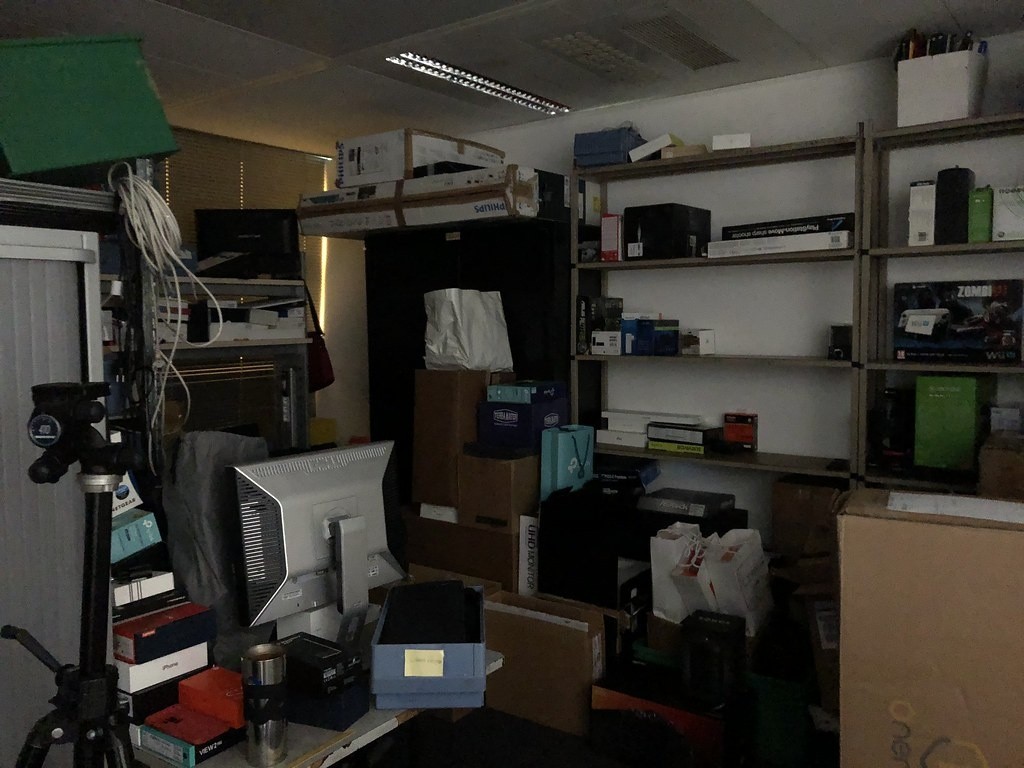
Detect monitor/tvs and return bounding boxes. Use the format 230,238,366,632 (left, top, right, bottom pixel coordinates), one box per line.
225,438,411,653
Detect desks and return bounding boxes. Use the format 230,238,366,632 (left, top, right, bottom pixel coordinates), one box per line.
132,652,504,768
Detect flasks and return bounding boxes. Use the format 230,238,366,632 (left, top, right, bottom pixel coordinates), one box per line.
242,644,288,768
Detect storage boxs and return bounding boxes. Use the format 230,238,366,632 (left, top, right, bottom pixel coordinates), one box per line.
578,297,715,356
110,479,368,768
284,131,602,234
411,367,497,508
894,280,1024,367
372,582,486,710
488,375,585,402
485,590,603,739
401,510,520,592
573,130,648,166
915,375,1023,500
0,32,180,178
592,671,729,754
593,407,756,457
837,490,1024,768
624,203,711,260
896,53,988,128
455,452,538,533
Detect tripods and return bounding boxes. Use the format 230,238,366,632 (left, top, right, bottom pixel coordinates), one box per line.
0,381,136,768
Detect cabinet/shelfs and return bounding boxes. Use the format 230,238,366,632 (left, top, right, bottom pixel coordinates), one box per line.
368,224,599,575
865,113,1023,506
570,126,860,708
